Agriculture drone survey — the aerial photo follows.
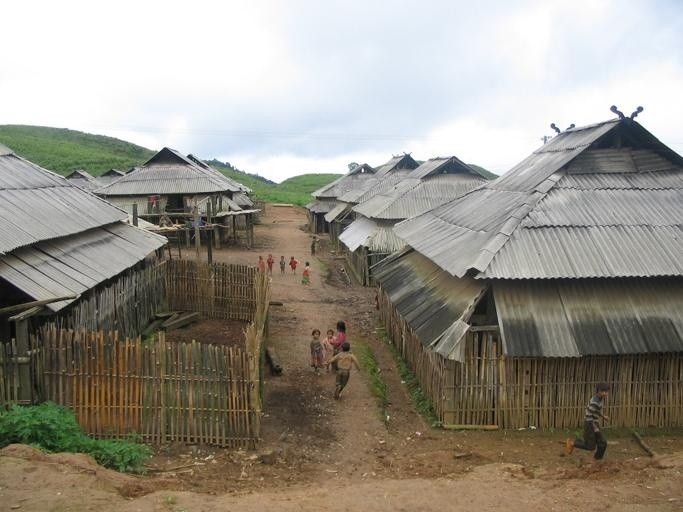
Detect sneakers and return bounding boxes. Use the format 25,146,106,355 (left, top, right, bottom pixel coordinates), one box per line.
566,438,575,454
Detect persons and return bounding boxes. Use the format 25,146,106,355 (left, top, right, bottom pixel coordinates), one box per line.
300,261,311,288
329,319,347,372
266,253,274,277
309,329,324,376
322,329,335,374
309,235,319,256
324,342,361,400
288,256,297,274
279,256,285,274
565,382,610,463
257,255,265,272
